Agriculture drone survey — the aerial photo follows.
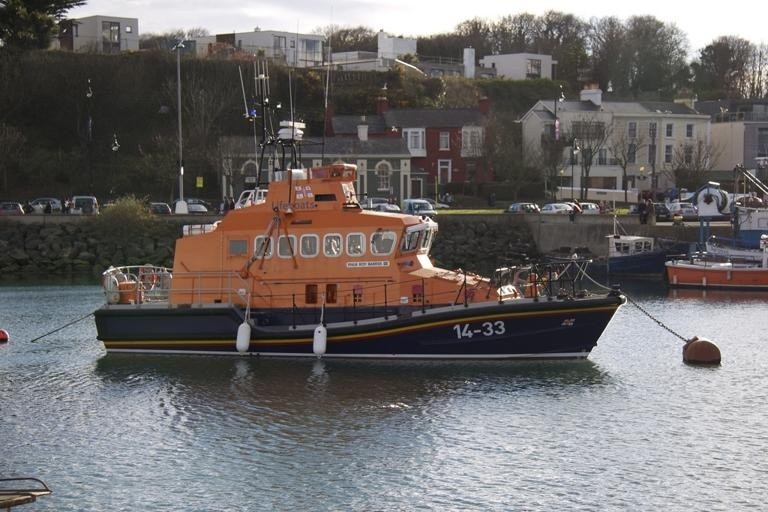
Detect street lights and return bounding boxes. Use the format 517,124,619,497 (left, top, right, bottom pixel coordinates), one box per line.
170,38,188,200
551,81,565,202
559,167,564,201
638,164,644,201
569,135,580,201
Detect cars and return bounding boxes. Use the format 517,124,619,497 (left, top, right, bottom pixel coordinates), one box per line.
503,187,697,224
0,196,209,216
359,194,450,216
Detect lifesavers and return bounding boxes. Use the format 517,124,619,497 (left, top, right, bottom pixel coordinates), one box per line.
139,267,156,289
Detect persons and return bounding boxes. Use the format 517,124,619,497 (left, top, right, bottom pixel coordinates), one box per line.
15,198,100,215
648,198,655,222
638,198,647,224
748,190,762,204
135,263,159,300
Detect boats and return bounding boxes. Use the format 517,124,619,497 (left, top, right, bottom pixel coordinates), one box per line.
703,237,763,262
662,152,768,292
92,43,630,367
543,201,693,285
